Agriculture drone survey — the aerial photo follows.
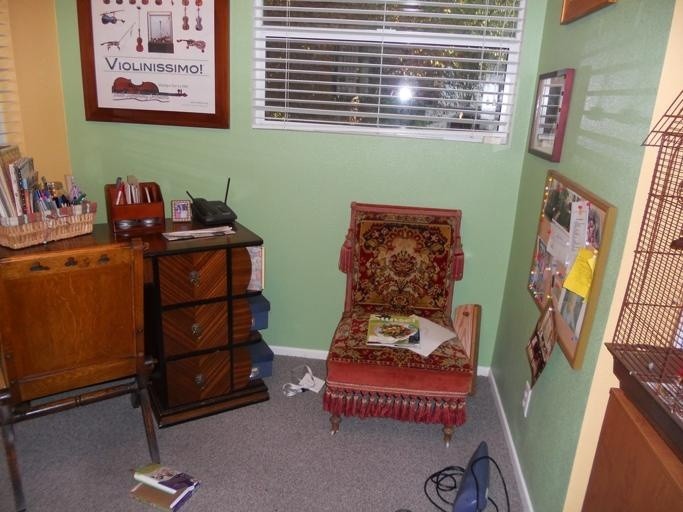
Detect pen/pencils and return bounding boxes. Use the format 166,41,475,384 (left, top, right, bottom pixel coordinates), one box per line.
115,177,122,203
115,182,124,206
33,176,70,217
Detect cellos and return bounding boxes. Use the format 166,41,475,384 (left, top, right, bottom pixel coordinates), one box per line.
112,78,188,97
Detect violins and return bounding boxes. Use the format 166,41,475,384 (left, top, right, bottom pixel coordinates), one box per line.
102,14,127,24
195,8,202,31
182,7,189,29
136,29,143,52
177,38,206,53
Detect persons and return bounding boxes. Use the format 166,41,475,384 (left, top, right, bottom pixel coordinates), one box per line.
177,205,187,219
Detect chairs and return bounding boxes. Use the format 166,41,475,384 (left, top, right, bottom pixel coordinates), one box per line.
322,201,473,447
2,239,160,512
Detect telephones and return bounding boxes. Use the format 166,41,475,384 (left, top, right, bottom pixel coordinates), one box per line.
186,178,238,227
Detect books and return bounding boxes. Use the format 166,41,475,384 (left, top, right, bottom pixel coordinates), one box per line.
0,145,39,227
145,186,153,204
365,313,420,348
114,174,141,206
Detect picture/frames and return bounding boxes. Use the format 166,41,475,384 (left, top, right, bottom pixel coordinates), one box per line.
528,67,575,164
76,0,231,130
527,169,618,371
172,200,191,223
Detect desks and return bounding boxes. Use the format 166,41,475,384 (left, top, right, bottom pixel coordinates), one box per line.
1,223,271,427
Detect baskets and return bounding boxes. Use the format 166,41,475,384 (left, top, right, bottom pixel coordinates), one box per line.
0,200,97,249
